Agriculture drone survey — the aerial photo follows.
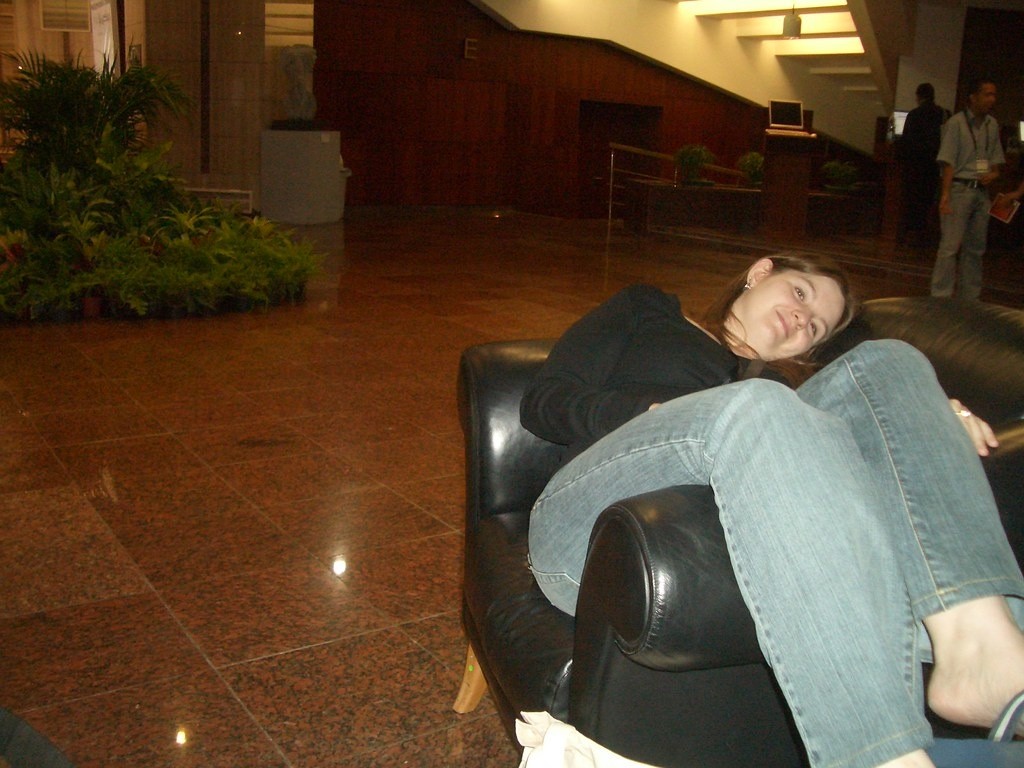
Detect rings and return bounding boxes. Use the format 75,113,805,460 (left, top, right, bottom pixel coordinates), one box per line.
956,410,970,418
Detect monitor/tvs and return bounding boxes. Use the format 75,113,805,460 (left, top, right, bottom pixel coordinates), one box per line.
894,110,909,136
768,100,804,130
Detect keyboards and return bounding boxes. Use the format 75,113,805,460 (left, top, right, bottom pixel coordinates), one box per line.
766,128,809,137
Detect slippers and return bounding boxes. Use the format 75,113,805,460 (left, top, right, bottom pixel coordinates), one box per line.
925,689,1024,768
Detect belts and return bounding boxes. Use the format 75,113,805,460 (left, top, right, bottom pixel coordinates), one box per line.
952,178,981,189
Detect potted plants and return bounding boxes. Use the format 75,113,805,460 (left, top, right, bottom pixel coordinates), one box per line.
51,215,105,320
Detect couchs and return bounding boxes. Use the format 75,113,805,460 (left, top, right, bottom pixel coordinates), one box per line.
457,296,1024,766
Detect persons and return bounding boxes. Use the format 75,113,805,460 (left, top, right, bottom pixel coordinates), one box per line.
932,78,1006,312
891,82,952,248
516,249,1024,768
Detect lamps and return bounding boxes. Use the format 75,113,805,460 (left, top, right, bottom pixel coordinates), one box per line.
782,3,802,40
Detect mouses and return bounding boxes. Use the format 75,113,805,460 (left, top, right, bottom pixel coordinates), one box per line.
811,133,817,138
